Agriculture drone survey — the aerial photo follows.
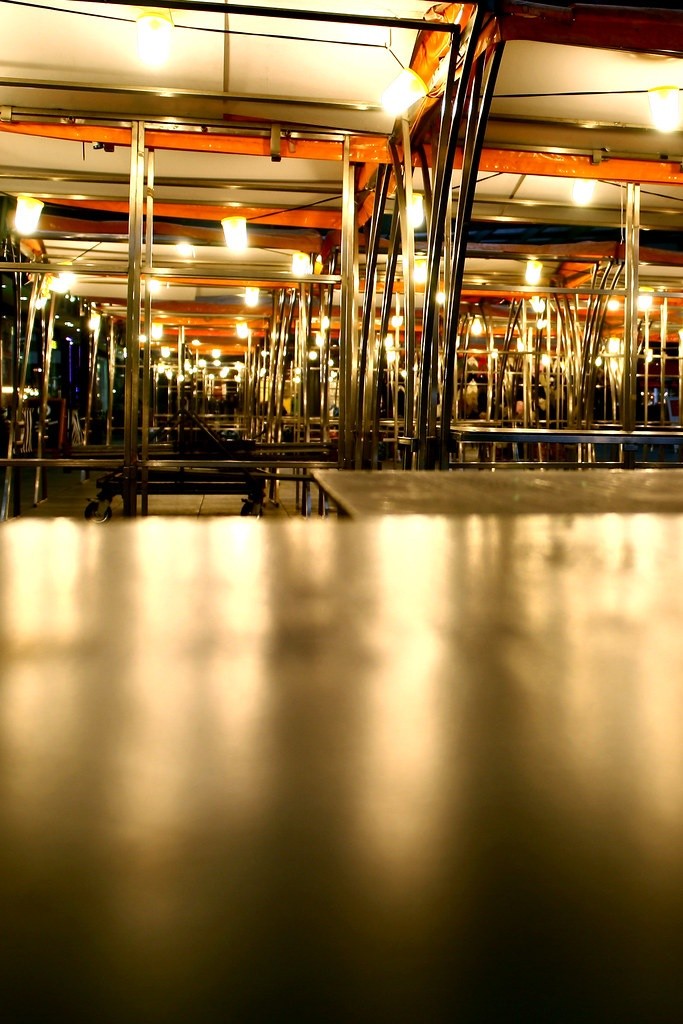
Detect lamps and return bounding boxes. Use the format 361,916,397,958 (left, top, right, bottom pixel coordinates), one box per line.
13,195,45,235
220,215,249,252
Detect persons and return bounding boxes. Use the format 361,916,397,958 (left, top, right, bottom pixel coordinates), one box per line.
208,392,240,423
